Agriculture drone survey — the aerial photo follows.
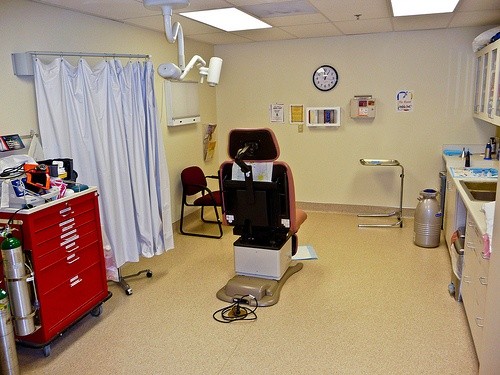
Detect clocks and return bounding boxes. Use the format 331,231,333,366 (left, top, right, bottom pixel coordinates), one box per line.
313,65,338,93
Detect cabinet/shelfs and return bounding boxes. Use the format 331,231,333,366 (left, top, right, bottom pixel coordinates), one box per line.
0,187,112,355
440,38,499,374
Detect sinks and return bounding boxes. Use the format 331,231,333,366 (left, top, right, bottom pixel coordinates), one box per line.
459,180,497,191
466,189,496,202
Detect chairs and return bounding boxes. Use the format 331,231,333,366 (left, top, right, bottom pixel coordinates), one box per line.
209,128,307,235
180,166,223,239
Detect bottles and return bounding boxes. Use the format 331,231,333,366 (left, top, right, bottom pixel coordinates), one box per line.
465,151,470,167
485,142,491,159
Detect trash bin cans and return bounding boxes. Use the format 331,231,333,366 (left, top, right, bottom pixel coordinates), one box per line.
448,226,465,302
